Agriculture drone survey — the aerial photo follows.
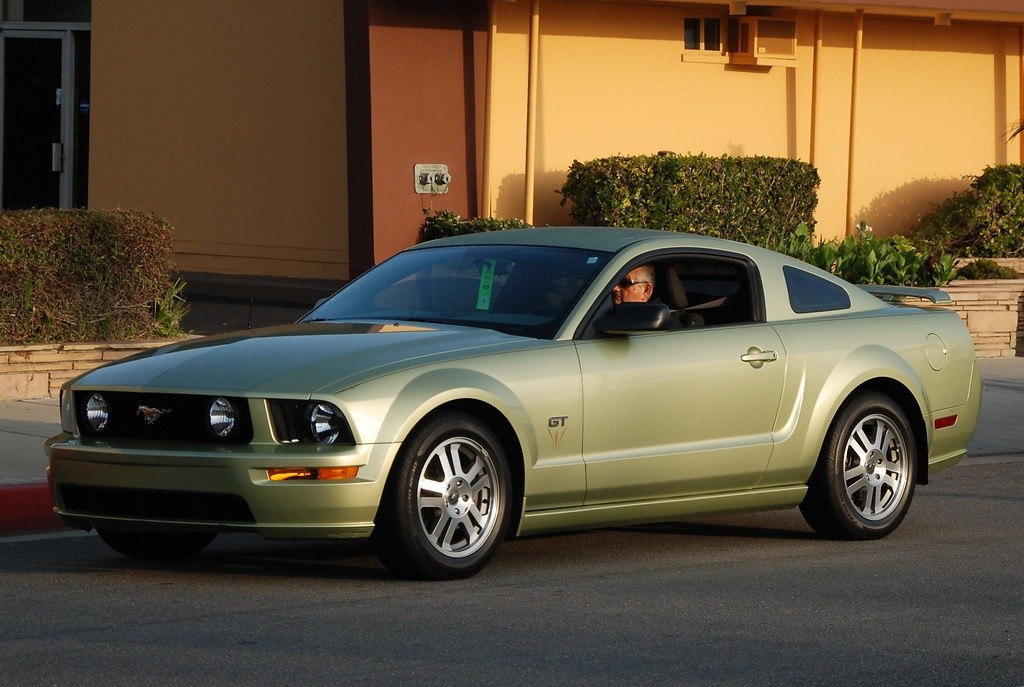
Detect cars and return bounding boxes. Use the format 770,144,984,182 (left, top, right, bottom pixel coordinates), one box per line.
42,222,984,583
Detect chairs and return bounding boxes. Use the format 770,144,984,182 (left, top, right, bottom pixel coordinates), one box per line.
647,266,705,332
491,267,563,321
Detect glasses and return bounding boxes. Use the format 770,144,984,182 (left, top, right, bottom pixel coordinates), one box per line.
619,277,649,288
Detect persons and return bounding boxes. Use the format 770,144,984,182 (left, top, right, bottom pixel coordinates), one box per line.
612,263,683,331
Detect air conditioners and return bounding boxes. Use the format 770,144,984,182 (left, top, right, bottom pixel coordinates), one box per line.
730,16,800,67
